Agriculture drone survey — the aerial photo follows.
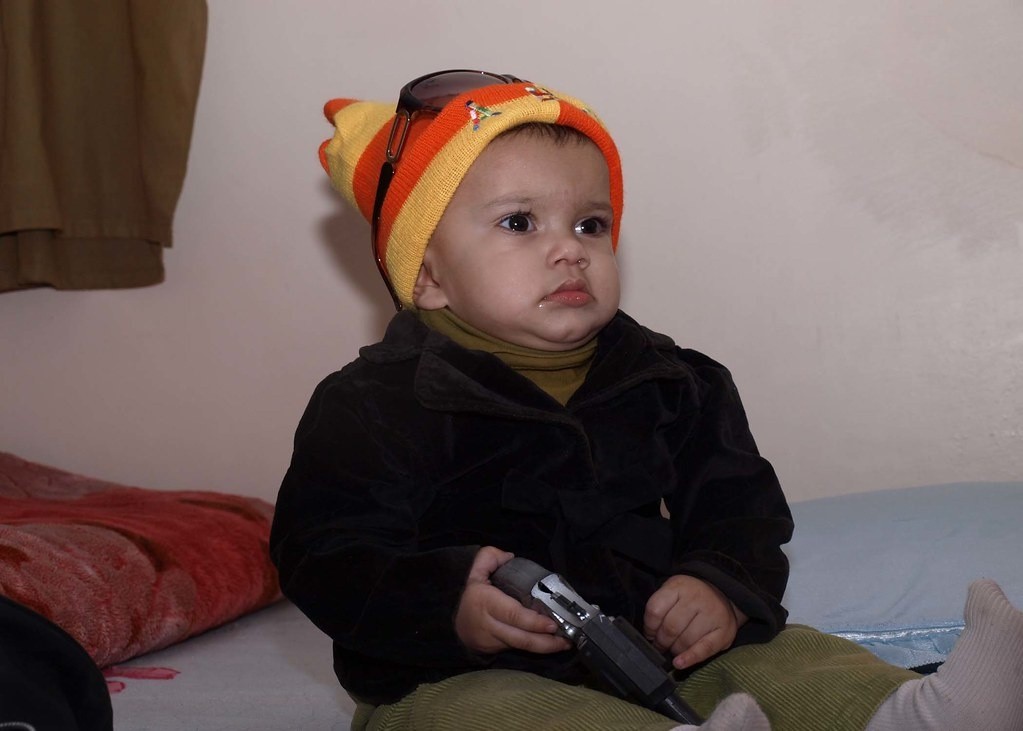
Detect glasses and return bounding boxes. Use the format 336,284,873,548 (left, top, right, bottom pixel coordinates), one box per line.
369,67,522,312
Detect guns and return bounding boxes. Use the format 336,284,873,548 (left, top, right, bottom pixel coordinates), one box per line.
490,558,712,728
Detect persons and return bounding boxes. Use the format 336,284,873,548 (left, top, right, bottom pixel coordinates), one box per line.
268,79,1023,731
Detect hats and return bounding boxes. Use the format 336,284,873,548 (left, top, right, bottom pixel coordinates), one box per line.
317,84,624,311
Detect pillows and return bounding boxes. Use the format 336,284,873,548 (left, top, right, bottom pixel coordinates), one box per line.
778,480,1023,671
